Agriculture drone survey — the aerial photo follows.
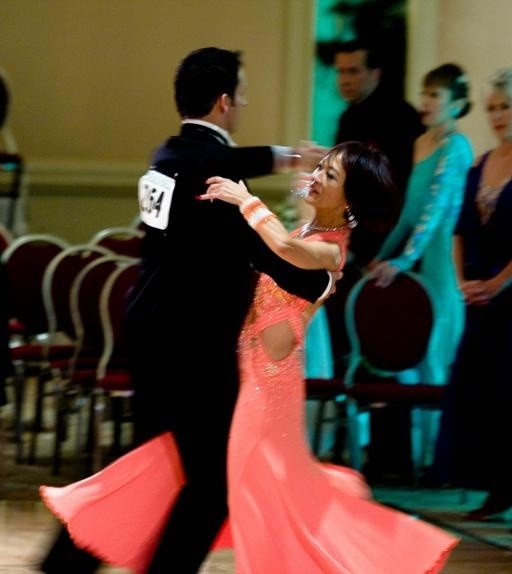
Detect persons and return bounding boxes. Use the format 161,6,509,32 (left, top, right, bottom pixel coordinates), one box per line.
428,69,512,522
326,37,427,463
38,140,462,574
345,63,474,474
35,49,345,574
0,78,31,238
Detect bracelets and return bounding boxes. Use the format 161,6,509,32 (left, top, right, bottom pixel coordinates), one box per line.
291,172,317,200
239,195,277,233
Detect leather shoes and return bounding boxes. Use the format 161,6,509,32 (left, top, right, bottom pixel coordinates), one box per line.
466,488,512,517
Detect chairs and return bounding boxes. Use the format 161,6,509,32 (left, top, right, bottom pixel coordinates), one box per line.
1,209,147,495
310,267,440,481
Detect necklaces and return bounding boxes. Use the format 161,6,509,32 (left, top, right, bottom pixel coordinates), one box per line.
300,223,347,237
